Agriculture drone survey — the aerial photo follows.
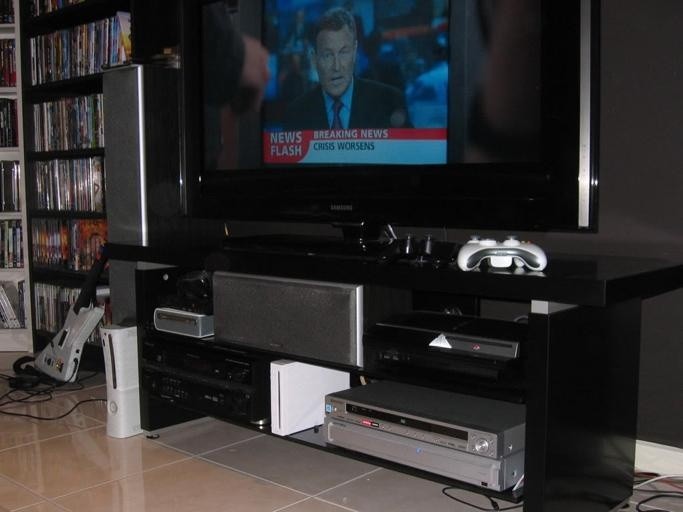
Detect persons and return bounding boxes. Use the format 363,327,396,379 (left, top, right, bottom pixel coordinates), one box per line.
187,2,451,176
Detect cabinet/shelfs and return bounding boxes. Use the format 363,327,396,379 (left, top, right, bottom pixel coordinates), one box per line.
0,0,33,353
20,0,182,372
102,235,683,512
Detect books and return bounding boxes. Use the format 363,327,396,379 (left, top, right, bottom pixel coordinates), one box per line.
34,93,104,152
30,11,132,86
33,156,104,213
31,1,82,17
0,1,25,329
32,218,110,272
34,282,113,347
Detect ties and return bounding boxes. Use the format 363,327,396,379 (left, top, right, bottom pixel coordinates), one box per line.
330,100,344,129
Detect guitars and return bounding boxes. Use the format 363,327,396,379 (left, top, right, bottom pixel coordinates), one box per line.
33,243,108,383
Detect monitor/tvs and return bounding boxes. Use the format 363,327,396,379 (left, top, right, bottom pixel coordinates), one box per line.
174,0,603,265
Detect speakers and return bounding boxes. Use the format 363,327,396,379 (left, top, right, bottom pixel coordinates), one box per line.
102,65,179,249
210,271,364,369
